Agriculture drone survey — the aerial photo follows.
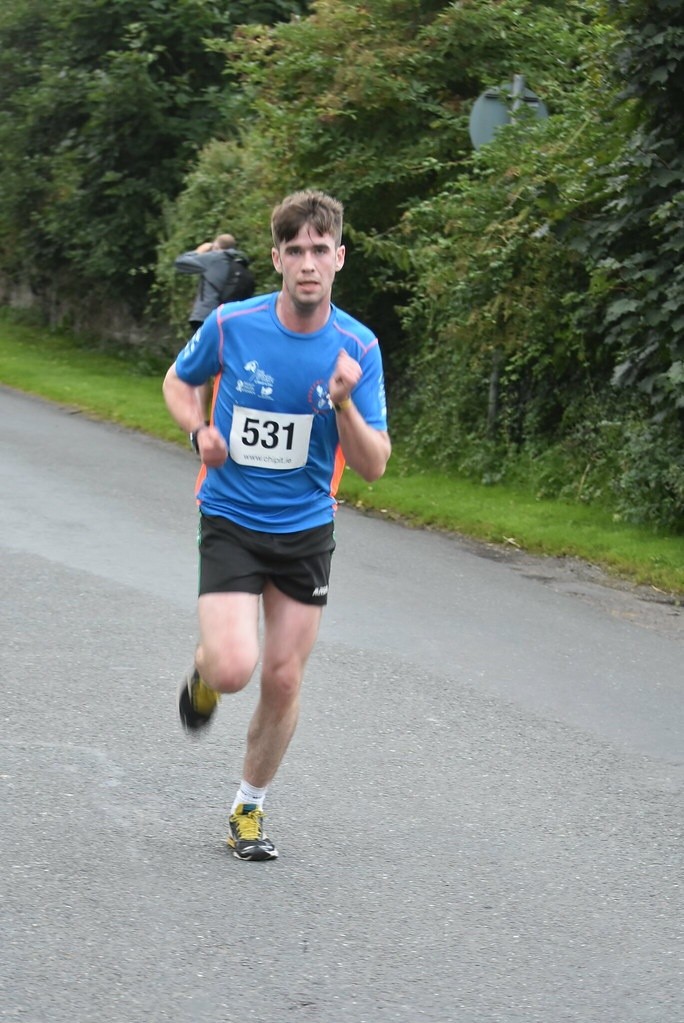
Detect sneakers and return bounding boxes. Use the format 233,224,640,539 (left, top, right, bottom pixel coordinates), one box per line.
225,803,279,861
176,667,220,736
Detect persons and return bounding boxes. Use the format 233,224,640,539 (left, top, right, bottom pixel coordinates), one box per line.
161,190,392,864
175,234,252,425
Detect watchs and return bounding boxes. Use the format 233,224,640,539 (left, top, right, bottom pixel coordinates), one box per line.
190,426,207,456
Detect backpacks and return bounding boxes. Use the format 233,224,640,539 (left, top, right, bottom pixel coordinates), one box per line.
200,250,256,306
